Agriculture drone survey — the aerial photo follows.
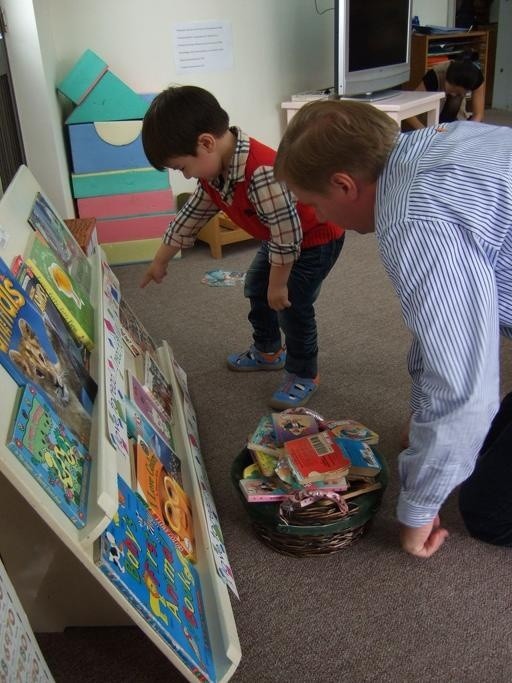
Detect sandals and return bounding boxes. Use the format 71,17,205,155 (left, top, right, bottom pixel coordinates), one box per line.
227,343,286,372
270,368,319,412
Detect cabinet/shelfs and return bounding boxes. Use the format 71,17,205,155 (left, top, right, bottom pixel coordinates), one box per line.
63,218,98,256
401,31,489,131
0,164,241,683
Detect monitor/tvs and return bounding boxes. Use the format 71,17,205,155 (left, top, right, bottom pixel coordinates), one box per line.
334,0,413,102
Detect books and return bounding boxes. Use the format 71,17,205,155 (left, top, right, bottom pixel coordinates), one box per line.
238,405,387,510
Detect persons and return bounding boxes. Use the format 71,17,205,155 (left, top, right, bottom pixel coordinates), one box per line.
272,98,511,560
137,84,346,416
402,58,488,131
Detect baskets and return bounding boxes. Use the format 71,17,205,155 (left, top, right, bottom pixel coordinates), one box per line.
229,442,390,559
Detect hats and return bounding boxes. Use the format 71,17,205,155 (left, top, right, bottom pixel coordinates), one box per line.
136,444,165,524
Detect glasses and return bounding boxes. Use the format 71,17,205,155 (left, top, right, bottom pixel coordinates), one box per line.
163,477,182,533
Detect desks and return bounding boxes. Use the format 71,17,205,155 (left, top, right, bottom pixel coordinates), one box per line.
281,91,446,135
